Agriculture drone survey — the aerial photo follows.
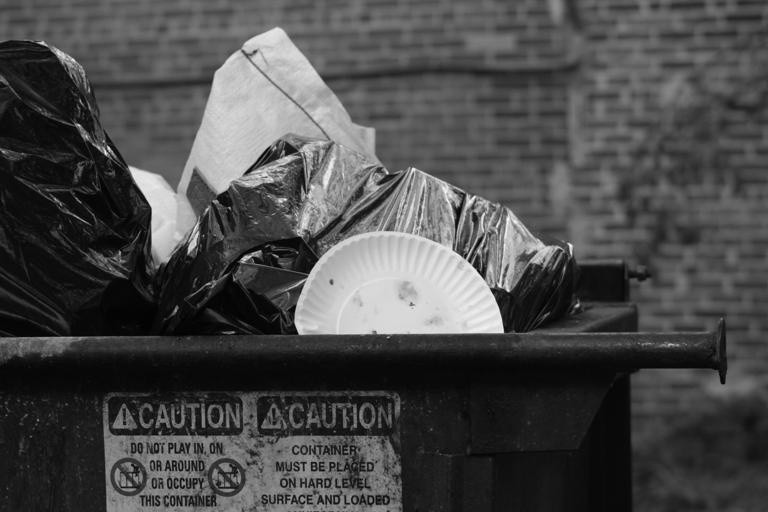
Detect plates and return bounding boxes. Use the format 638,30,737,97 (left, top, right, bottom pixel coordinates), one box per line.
291,229,505,334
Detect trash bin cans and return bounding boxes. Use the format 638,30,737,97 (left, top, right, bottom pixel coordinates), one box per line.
0,252,730,512
